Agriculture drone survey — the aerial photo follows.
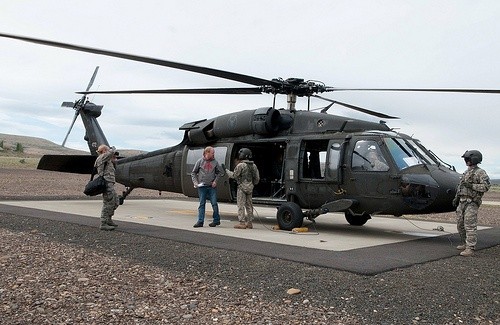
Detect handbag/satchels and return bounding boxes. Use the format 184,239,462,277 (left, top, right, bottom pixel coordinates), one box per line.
84,176,107,196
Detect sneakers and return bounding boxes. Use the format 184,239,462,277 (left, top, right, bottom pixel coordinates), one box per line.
233,224,246,229
246,223,252,228
193,221,203,227
209,222,220,227
457,245,466,249
100,225,114,230
460,249,474,256
108,222,118,227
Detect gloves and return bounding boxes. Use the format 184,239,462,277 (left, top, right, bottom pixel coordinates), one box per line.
453,198,458,206
461,180,472,188
222,164,226,170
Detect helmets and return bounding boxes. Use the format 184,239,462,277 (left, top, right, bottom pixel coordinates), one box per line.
462,150,482,166
239,148,252,159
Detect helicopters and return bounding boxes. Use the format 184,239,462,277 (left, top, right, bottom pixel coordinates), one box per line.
1,31,500,230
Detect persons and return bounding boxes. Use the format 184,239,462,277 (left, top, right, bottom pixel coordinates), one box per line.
365,152,389,171
191,146,220,228
452,150,491,256
222,148,260,229
94,145,119,231
